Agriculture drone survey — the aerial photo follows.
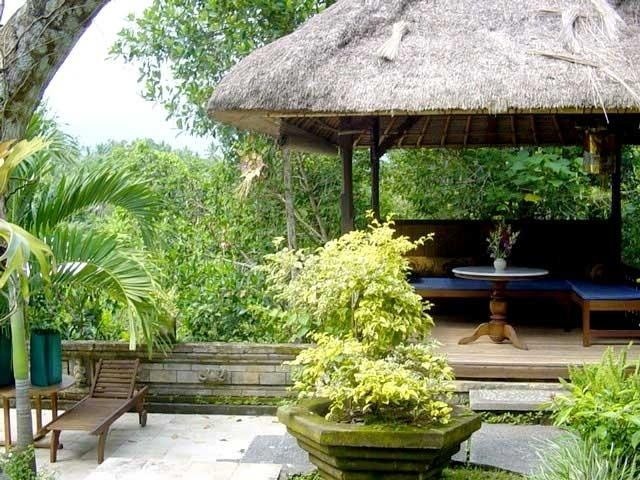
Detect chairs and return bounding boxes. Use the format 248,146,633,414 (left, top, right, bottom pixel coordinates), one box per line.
45,357,150,465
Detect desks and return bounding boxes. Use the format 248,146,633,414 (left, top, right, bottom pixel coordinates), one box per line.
451,265,551,351
0,373,76,453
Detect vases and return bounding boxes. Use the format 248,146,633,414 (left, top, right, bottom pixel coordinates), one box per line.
492,258,507,271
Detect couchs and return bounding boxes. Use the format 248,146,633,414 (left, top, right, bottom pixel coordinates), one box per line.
388,218,639,347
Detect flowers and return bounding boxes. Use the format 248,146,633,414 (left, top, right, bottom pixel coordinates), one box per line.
485,215,521,260
